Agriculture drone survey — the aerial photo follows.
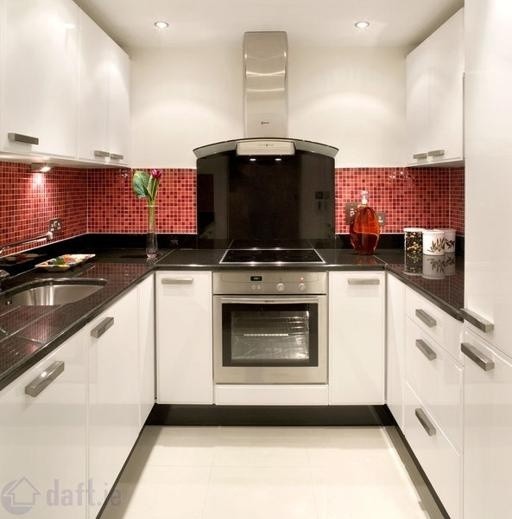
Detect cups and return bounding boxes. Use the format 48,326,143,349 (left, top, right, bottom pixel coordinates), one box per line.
405,252,456,280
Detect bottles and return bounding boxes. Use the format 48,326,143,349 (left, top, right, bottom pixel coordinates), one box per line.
404,227,456,254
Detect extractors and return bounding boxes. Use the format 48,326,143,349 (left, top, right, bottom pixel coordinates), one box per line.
192,29,339,159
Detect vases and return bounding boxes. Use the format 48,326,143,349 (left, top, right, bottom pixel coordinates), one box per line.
144,205,160,258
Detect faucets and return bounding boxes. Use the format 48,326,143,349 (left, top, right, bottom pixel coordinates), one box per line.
0,231,53,256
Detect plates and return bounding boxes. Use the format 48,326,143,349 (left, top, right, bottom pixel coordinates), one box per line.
33,254,95,270
33,263,96,279
0,253,43,267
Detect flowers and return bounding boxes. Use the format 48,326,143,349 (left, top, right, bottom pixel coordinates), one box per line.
133,168,164,230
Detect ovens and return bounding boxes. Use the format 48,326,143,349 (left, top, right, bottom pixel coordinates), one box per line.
212,270,328,404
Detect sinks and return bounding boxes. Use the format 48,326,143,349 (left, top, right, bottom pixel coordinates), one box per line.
0,277,106,305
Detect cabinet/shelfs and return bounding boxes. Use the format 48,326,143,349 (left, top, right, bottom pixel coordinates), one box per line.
328,270,385,410
0,274,152,519
461,324,511,519
81,11,128,165
154,272,212,407
0,2,80,160
460,2,512,356
404,6,464,168
383,272,461,517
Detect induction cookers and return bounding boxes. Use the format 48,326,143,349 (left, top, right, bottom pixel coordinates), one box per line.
218,248,327,264
